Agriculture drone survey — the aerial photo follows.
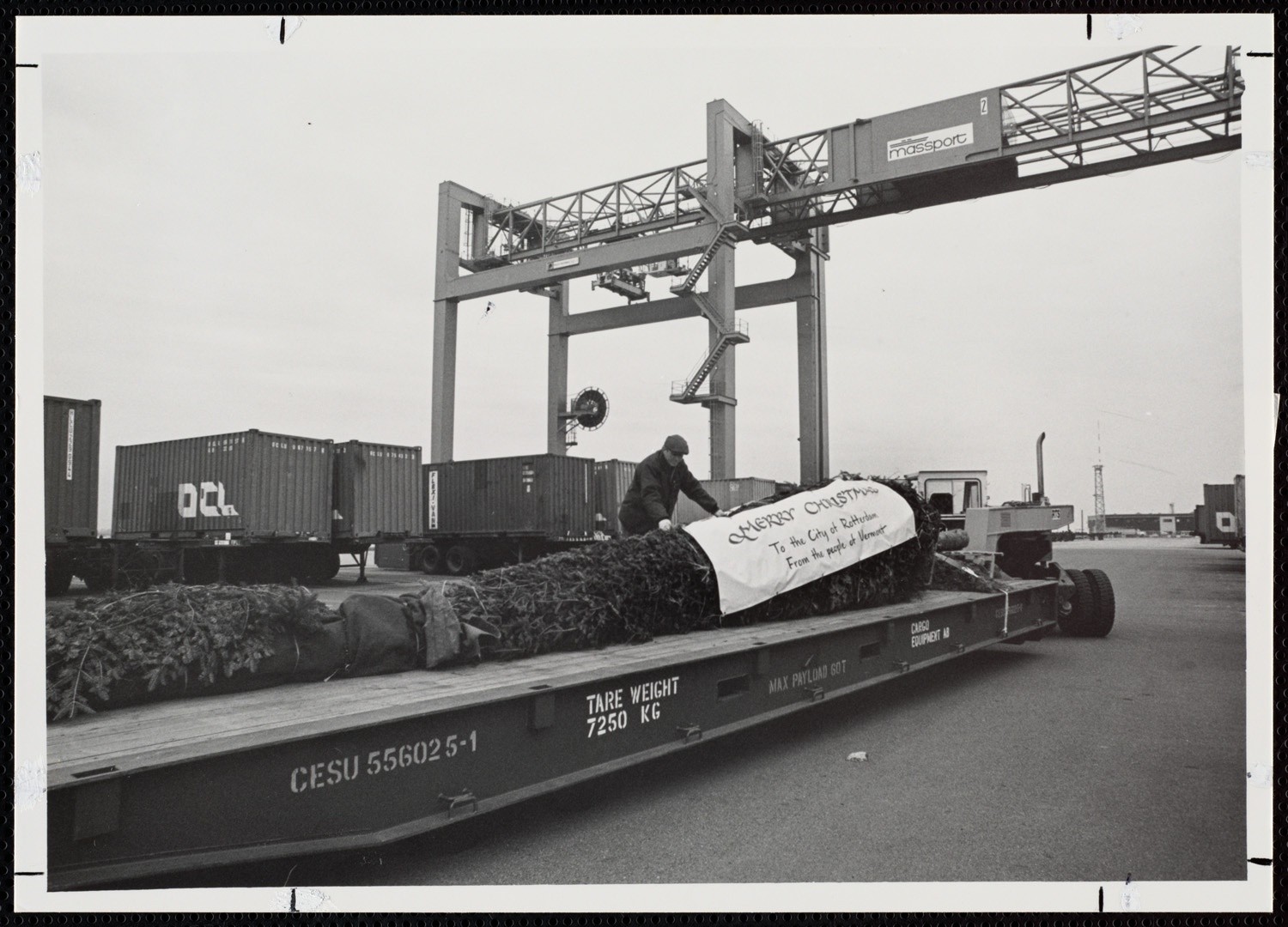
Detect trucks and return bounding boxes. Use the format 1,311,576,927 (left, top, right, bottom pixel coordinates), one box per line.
47,431,1119,882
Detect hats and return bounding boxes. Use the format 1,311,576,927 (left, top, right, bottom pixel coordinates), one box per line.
665,435,689,454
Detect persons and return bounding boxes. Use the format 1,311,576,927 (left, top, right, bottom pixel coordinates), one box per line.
617,435,727,536
